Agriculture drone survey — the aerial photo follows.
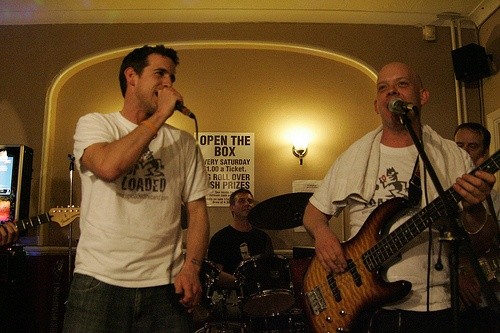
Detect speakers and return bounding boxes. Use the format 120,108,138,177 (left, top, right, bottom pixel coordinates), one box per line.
451,43,491,82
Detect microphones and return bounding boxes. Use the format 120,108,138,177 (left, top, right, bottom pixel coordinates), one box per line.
175,101,196,120
388,98,418,115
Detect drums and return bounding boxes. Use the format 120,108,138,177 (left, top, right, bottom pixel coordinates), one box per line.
233,253,296,318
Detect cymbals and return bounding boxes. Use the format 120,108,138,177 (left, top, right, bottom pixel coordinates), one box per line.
246,192,314,231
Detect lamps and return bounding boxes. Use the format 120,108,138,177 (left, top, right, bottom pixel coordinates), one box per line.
293,146,308,165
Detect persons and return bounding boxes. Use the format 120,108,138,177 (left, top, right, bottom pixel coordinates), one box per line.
0,218,19,247
61,43,214,333
303,61,500,333
207,186,277,300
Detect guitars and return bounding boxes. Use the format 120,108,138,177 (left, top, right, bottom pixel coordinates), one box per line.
302,148,500,333
0,205,81,236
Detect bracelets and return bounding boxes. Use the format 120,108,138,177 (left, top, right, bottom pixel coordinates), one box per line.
139,119,157,138
460,207,488,234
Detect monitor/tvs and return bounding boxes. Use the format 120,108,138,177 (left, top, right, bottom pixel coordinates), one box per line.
0,156,14,196
